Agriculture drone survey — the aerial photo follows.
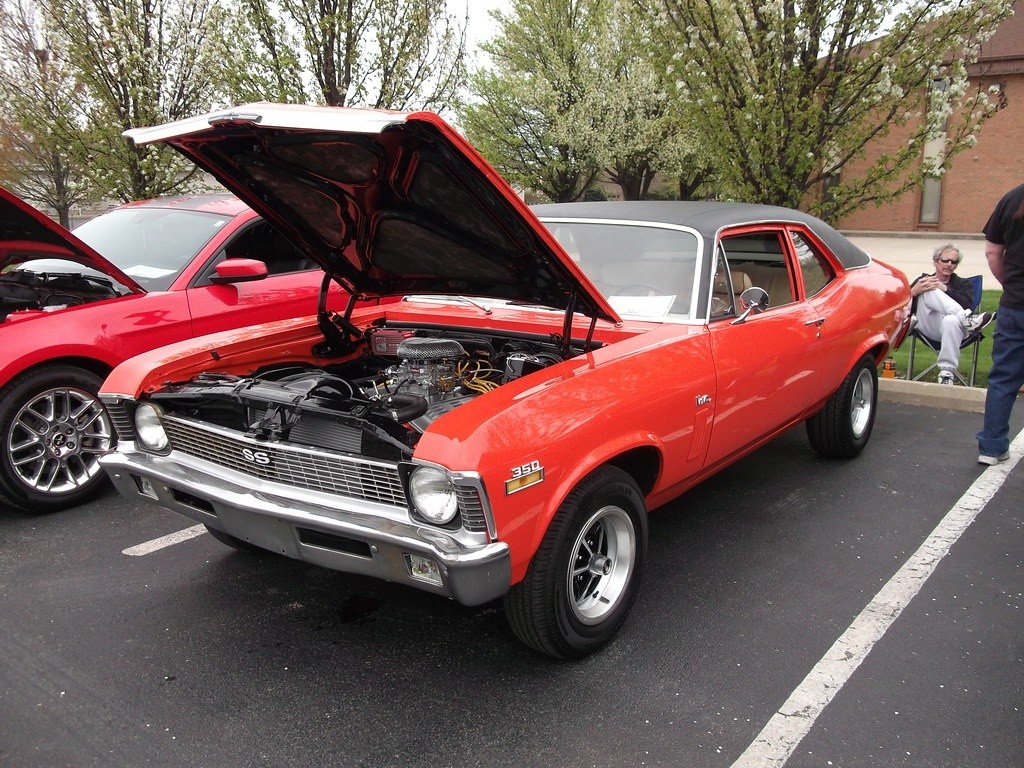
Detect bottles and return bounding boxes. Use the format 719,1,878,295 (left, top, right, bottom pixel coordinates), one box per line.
882,355,895,378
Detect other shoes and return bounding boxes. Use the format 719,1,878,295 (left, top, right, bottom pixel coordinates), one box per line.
977,450,1009,465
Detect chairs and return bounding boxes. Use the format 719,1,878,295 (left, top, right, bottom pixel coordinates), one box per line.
586,261,649,305
907,274,998,385
265,228,306,272
683,270,755,318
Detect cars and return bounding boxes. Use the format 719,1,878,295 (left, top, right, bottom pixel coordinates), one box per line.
0,184,407,515
96,102,912,658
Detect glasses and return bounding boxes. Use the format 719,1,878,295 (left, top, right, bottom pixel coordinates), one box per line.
936,257,959,264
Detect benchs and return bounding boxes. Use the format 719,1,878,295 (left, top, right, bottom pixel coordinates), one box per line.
635,257,815,312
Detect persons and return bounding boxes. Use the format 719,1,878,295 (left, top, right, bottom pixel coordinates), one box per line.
975,183,1024,466
910,243,996,387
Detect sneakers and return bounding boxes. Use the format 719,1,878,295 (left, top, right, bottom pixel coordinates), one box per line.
938,374,953,386
963,308,991,337
